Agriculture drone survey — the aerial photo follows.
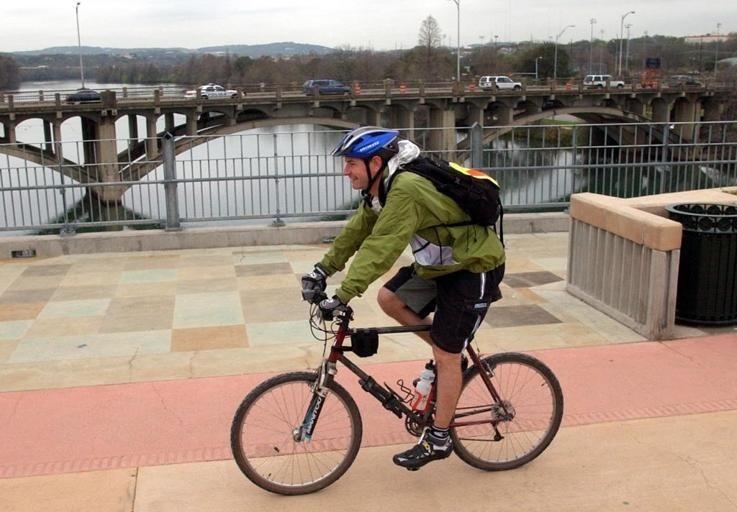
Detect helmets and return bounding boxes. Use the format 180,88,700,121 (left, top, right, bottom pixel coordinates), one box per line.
329,126,399,157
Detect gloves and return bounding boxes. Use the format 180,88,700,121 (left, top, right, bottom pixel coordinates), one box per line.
318,297,342,322
301,269,326,291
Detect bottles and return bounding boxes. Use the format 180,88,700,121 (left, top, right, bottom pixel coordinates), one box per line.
410,360,436,411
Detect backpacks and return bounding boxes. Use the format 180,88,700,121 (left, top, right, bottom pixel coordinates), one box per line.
378,151,501,228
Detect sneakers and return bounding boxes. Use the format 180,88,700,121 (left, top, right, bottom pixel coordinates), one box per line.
393,427,453,467
414,356,469,389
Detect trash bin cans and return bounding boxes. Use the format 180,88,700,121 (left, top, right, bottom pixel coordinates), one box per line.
664,201,737,326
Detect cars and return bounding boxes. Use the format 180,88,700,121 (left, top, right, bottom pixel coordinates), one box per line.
65,89,100,100
667,75,704,88
632,78,660,89
301,79,352,96
183,83,246,100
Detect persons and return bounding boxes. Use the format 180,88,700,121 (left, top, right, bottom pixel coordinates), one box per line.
300,124,507,469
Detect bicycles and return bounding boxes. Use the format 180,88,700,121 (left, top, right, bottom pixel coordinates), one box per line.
226,283,564,495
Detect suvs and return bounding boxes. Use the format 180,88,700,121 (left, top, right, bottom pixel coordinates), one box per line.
478,75,522,93
582,75,624,90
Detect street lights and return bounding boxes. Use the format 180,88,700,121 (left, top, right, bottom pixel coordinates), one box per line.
74,1,85,88
713,22,721,78
533,8,646,77
